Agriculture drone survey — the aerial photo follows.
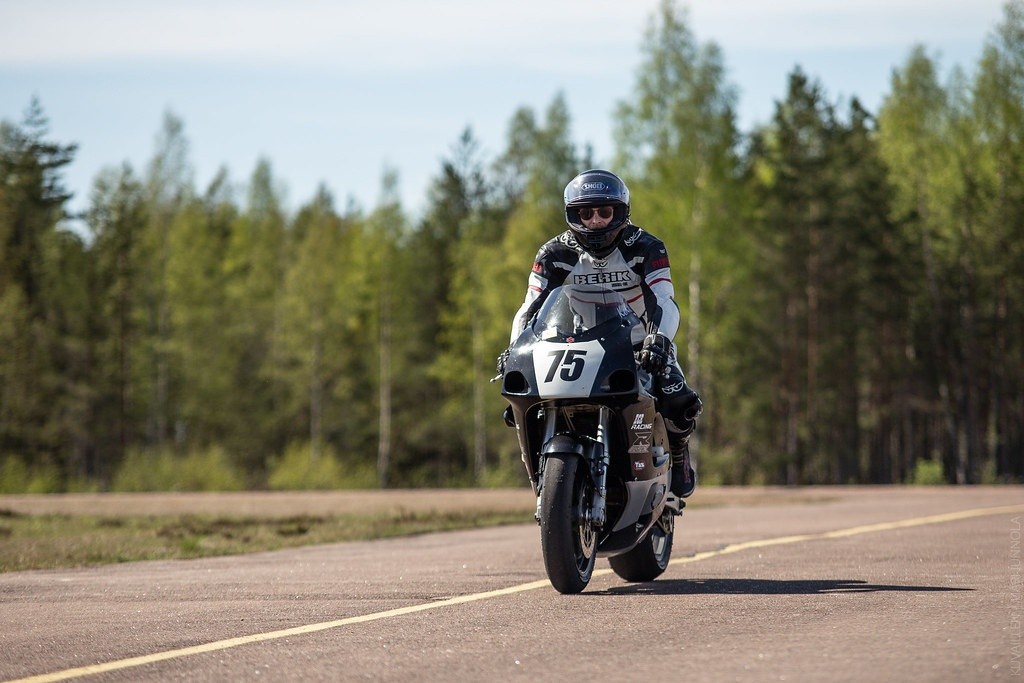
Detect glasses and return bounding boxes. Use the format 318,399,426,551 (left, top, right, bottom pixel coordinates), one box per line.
577,208,614,220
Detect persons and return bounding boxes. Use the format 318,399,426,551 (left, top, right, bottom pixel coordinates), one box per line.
496,169,704,499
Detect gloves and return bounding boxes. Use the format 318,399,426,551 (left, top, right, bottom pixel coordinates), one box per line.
637,333,675,378
497,346,512,379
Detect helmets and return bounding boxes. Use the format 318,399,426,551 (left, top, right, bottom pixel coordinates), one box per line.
563,169,630,256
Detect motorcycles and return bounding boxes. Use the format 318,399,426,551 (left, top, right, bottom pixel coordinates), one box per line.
488,284,688,596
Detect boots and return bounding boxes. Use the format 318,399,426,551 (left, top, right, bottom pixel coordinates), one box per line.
664,417,697,498
